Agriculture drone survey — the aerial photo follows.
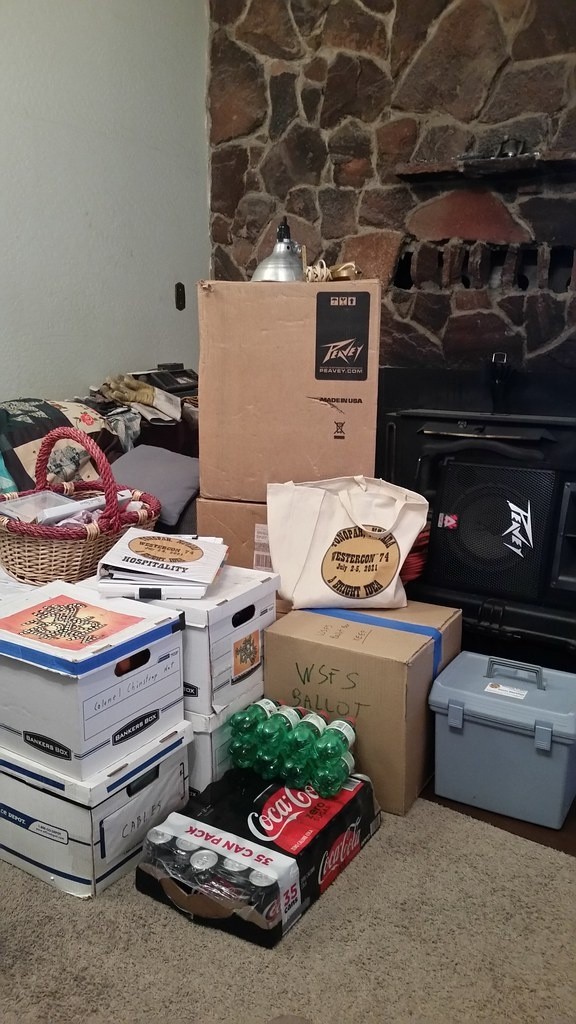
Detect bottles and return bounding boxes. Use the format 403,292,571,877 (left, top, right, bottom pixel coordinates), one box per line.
227,697,357,799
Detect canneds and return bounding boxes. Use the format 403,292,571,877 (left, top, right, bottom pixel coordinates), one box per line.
142,829,281,923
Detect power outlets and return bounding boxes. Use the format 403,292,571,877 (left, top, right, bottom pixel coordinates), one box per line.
175,282,185,311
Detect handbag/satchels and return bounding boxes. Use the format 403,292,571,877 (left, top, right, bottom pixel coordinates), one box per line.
266,475,429,610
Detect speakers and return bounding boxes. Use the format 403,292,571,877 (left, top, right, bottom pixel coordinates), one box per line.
423,456,557,604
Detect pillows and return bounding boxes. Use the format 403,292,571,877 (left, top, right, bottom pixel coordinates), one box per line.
103,444,200,527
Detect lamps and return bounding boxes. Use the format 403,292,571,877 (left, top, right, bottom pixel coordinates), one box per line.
248,214,307,283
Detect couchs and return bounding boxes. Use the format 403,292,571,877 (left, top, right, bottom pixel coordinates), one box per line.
0,395,200,605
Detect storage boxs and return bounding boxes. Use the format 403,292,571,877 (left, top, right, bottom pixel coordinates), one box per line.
263,594,465,817
183,683,263,803
73,562,282,714
0,718,195,902
0,580,188,781
196,496,294,615
403,581,576,673
196,277,383,499
426,650,576,831
135,762,384,948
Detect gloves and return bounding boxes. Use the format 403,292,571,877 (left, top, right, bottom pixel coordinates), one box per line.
100,373,182,425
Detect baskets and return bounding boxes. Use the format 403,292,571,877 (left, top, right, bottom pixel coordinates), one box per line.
0,426,161,586
